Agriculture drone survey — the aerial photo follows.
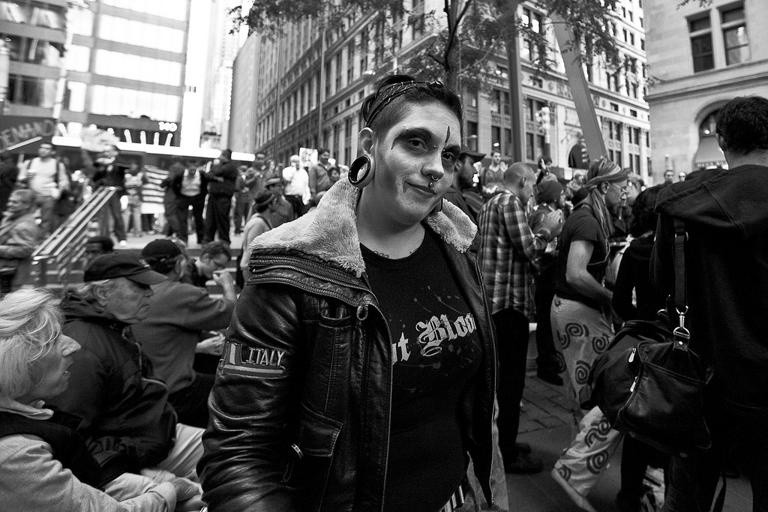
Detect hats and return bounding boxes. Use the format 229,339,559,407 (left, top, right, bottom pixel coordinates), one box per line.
83,252,168,286
535,180,563,203
265,174,281,187
582,157,631,187
460,143,486,162
254,189,275,210
141,239,181,265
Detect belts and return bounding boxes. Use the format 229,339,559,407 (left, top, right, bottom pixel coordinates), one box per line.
439,485,465,512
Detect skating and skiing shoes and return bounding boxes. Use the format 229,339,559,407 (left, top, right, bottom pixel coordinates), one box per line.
536,370,563,386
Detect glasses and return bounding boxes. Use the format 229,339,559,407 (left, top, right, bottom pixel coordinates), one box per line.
211,258,225,269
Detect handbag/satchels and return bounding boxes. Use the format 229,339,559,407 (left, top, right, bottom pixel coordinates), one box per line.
587,215,715,446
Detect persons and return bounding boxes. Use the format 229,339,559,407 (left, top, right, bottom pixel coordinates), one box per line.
445,144,487,225
477,161,564,476
663,169,675,185
326,165,341,190
678,171,688,182
196,73,508,512
481,149,509,189
527,179,568,387
281,154,312,218
565,172,585,200
48,251,207,494
122,238,237,414
84,235,114,265
0,284,205,512
246,176,293,228
179,240,232,288
244,151,266,209
649,95,768,512
16,141,66,238
308,148,334,200
549,154,629,512
233,164,247,234
0,188,42,294
612,184,670,324
202,150,239,245
173,158,209,244
240,189,279,285
159,156,184,243
87,144,130,242
121,159,149,238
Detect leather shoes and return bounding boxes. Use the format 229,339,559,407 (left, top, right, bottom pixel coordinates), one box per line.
551,469,595,511
504,455,542,473
514,443,531,455
